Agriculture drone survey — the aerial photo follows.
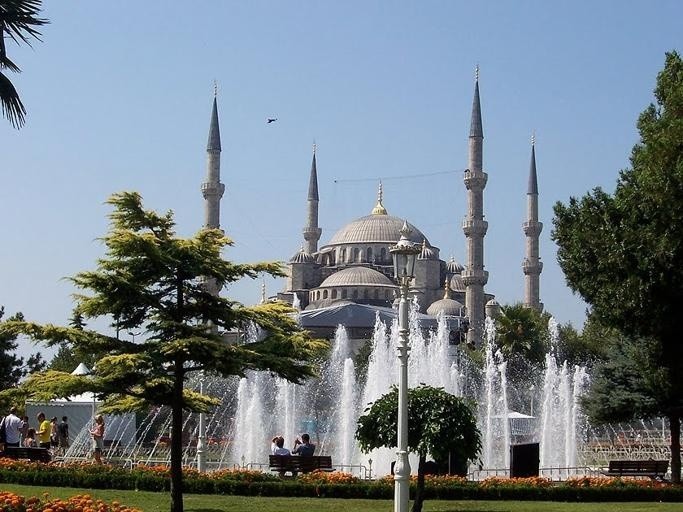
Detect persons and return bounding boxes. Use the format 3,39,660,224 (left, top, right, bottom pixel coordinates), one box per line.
291,433,315,479
18,415,27,447
0,406,23,447
270,435,291,478
21,428,36,447
50,416,59,451
58,415,68,455
33,412,51,451
87,414,105,466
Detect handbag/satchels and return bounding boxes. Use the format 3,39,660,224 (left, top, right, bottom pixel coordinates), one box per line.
0,416,7,443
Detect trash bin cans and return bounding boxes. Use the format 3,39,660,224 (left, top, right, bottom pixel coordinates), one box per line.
510,443,539,478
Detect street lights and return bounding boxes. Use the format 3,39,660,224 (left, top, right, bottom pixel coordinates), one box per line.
128,332,141,343
387,219,422,512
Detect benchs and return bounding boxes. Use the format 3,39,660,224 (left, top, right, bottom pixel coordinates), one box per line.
269,455,336,472
102,440,120,456
7,446,51,463
600,460,669,482
